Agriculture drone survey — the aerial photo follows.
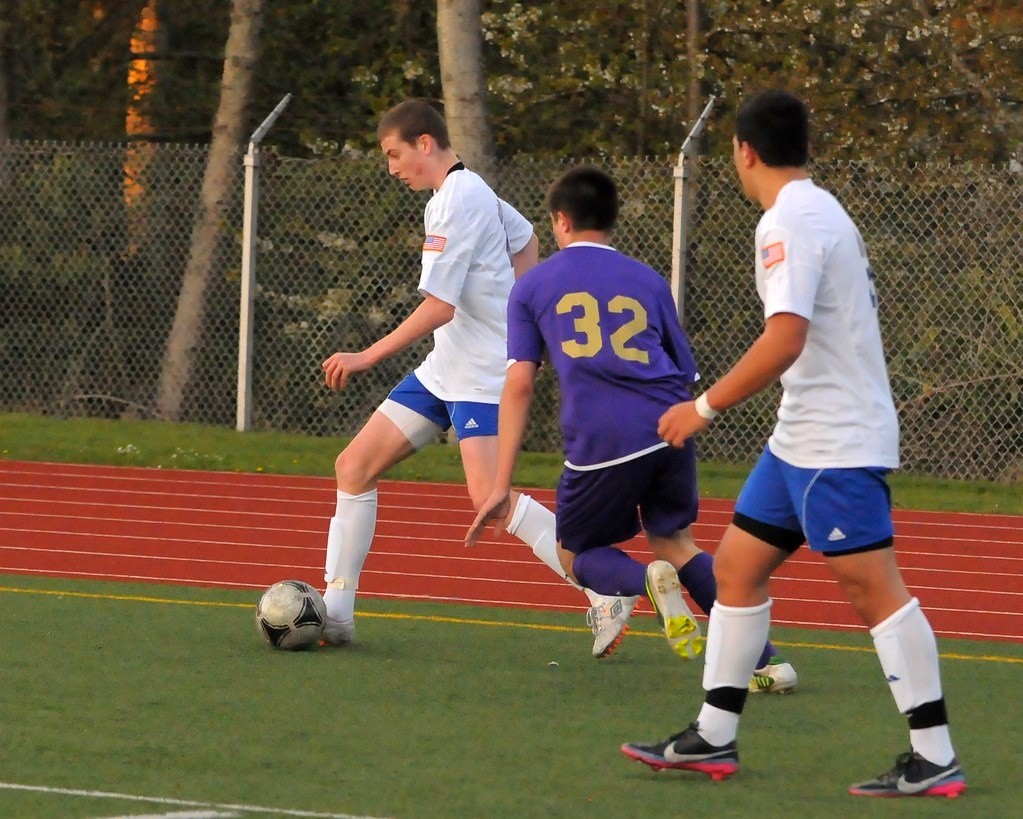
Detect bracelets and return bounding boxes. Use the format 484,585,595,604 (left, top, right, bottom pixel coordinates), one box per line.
695,390,718,421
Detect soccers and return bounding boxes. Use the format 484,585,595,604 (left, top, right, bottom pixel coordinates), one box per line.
254,579,327,651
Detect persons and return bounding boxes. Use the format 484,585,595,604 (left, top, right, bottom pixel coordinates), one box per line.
318,97,644,658
619,88,966,798
466,164,800,697
